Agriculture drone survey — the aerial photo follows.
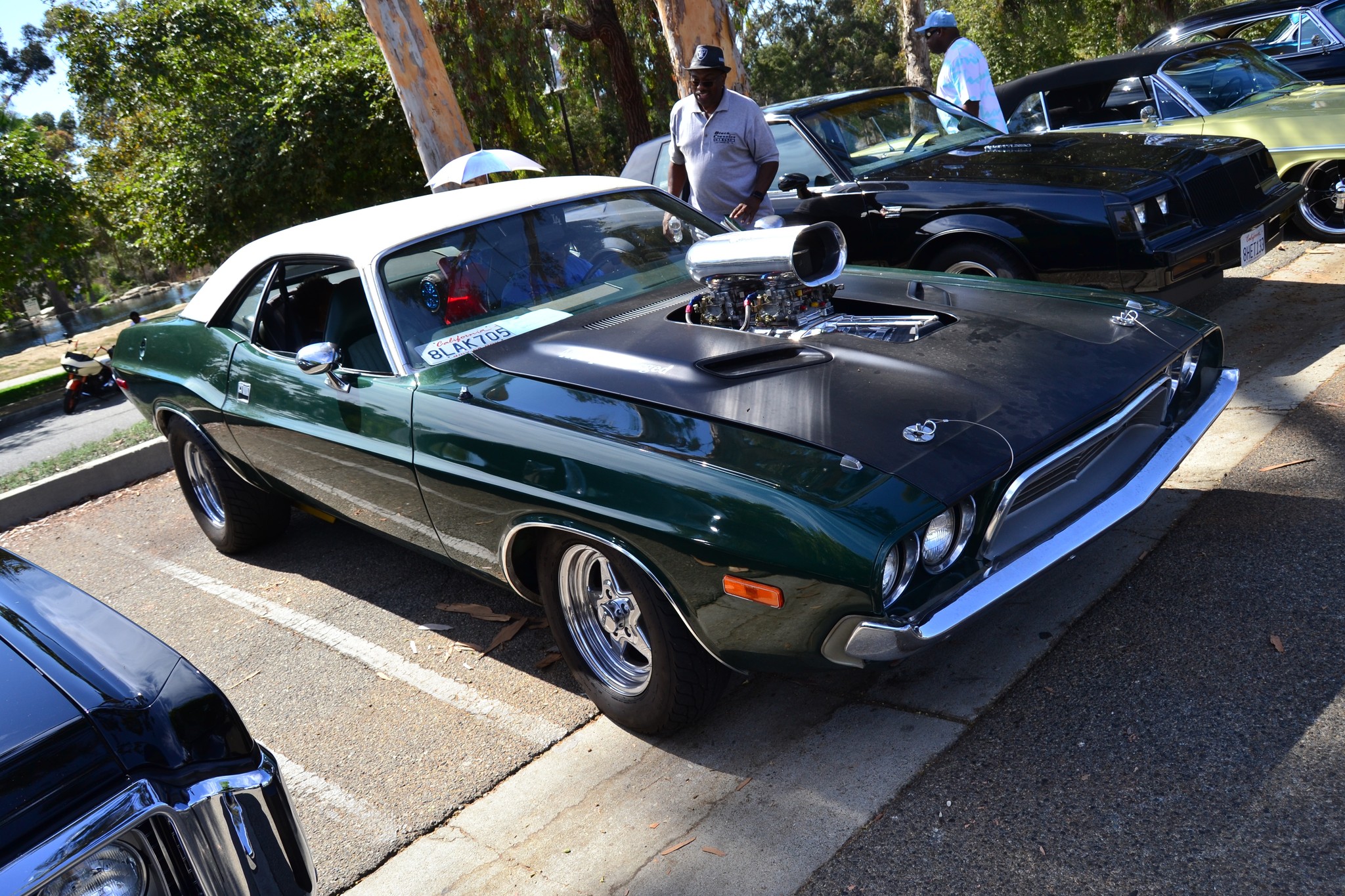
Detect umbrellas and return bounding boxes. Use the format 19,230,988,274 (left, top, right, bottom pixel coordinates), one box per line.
425,137,547,187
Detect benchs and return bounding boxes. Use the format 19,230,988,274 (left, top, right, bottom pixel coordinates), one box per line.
1025,99,1156,131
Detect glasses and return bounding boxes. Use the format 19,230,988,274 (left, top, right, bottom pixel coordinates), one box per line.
687,71,723,88
924,27,940,40
546,232,578,251
131,316,137,321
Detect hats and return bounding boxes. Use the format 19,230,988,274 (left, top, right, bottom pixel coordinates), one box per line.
682,45,732,74
914,8,958,32
531,206,566,226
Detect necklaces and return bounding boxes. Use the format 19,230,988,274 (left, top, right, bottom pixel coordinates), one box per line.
946,36,962,50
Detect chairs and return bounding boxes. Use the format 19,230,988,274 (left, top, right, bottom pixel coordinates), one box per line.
326,276,418,371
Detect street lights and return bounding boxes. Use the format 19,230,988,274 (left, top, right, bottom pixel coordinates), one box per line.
553,86,580,176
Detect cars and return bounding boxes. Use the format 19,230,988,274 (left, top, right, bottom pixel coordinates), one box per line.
849,37,1345,245
1103,0,1345,121
605,83,1310,311
0,547,322,896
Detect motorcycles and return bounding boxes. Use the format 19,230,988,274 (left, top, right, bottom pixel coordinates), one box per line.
60,332,121,415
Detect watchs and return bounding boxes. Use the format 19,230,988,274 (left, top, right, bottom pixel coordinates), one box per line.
750,190,764,202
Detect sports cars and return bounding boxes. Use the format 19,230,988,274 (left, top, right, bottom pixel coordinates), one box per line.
107,177,1239,742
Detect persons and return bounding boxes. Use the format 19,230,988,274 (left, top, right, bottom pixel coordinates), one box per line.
914,10,1010,135
292,273,333,354
662,45,779,246
500,219,605,308
129,312,148,325
